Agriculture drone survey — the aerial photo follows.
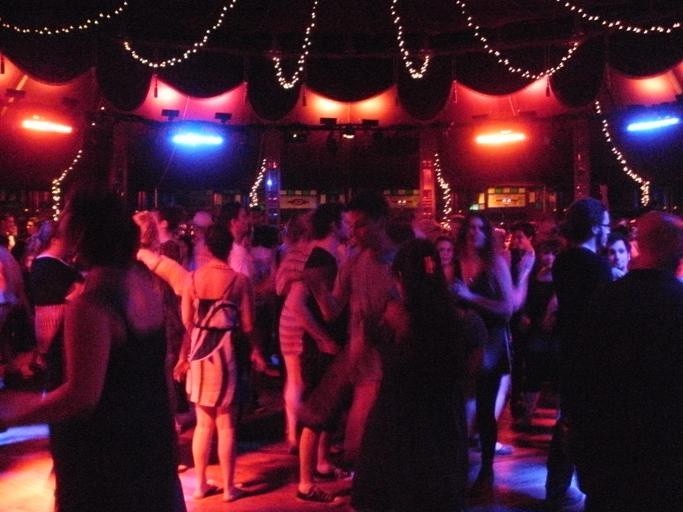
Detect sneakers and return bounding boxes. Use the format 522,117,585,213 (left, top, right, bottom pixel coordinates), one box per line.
298,482,333,503
314,465,349,480
470,468,494,494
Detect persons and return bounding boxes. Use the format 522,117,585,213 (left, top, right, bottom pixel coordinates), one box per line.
0,180,682,512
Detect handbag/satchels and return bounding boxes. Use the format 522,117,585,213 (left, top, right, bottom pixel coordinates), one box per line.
173,359,189,382
